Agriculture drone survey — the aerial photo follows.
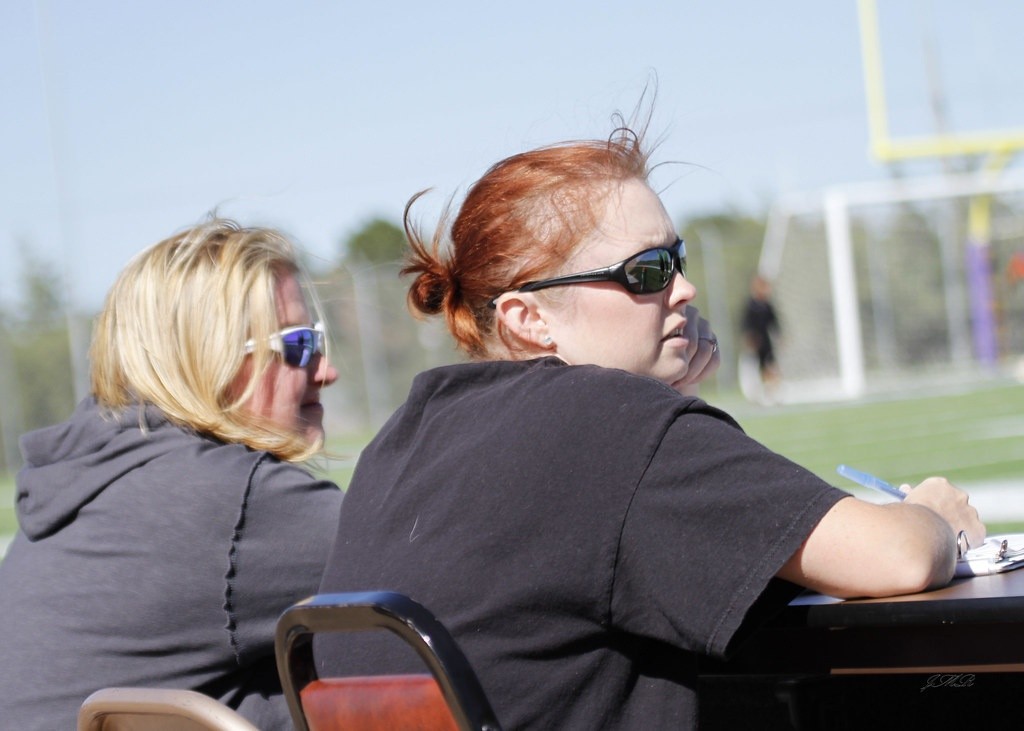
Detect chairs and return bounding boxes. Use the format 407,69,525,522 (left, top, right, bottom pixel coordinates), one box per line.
74,588,503,730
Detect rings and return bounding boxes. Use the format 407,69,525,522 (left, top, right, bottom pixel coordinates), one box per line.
698,337,717,352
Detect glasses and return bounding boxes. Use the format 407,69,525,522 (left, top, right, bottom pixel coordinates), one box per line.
244,321,326,368
488,239,687,309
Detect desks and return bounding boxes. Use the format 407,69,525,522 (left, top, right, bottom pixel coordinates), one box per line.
710,560,1023,731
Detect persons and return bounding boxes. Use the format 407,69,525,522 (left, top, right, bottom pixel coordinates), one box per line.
312,69,988,731
0,208,343,731
739,282,785,405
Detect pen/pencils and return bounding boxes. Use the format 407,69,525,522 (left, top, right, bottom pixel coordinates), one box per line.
837,466,908,501
